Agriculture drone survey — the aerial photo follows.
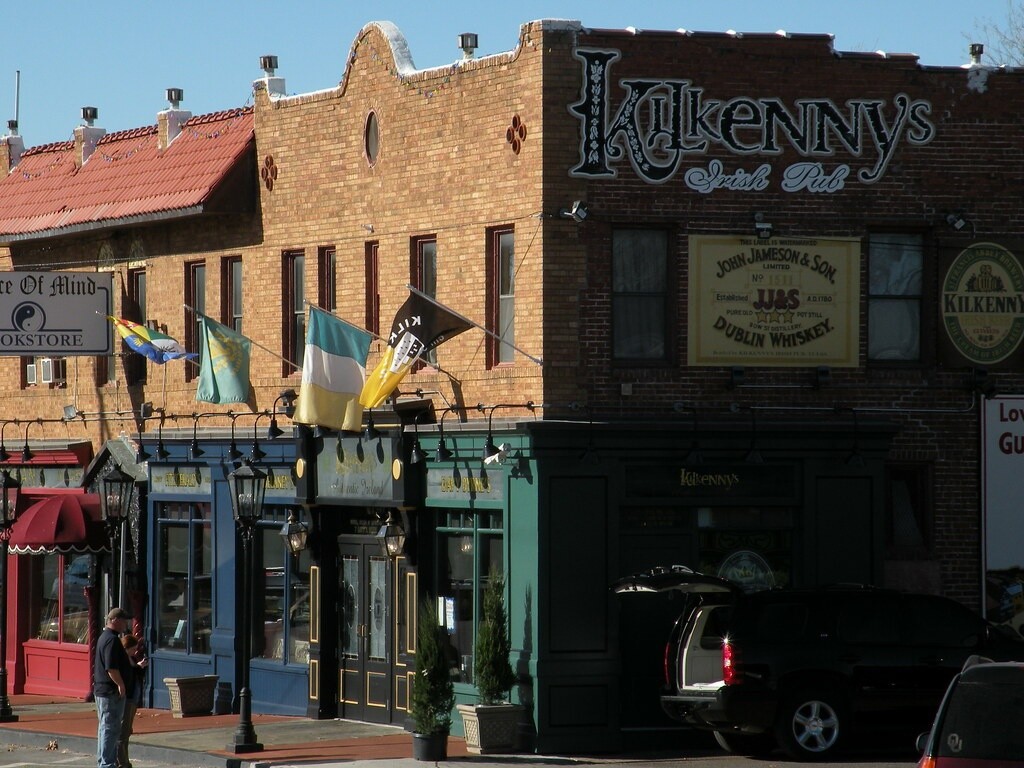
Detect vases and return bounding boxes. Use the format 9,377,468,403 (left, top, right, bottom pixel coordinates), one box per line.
163,675,220,719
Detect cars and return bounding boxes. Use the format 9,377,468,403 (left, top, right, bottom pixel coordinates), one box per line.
263,567,312,624
53,553,87,609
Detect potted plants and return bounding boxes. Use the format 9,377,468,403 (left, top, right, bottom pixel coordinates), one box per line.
404,591,456,761
453,561,525,755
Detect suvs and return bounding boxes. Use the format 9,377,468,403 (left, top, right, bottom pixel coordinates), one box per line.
609,563,1024,768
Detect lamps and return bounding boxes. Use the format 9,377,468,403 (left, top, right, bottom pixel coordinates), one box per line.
579,405,602,467
458,32,478,48
735,408,786,479
946,214,978,230
834,401,876,479
969,43,985,54
81,107,98,119
755,212,783,239
558,200,589,223
972,365,994,395
260,56,297,69
667,403,706,476
279,509,310,559
166,88,184,101
0,390,535,464
7,120,18,129
375,511,406,558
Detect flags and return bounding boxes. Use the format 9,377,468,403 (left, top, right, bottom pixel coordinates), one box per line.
359,292,475,408
291,307,372,432
194,311,252,403
110,316,199,365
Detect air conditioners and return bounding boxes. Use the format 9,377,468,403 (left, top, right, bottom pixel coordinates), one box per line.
27,365,37,384
41,358,65,384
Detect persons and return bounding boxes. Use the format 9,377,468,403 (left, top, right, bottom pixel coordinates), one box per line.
93,608,149,768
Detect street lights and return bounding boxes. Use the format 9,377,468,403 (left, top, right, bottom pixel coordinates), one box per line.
227,456,270,754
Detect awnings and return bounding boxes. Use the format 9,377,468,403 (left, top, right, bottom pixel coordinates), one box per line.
7,493,132,555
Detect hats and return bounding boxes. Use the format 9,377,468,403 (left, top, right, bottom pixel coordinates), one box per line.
107,608,133,620
